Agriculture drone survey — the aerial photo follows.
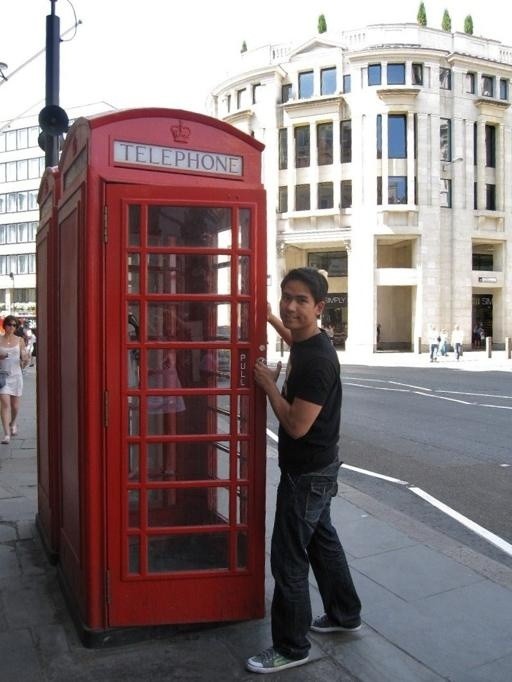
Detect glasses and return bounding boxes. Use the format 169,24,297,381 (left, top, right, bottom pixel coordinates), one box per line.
7,323,16,327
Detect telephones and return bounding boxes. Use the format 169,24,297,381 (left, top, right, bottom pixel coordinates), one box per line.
129,315,140,359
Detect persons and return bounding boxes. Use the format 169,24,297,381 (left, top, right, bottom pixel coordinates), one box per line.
0,315,31,443
323,324,335,346
474,322,486,348
428,324,464,363
377,323,381,343
247,267,361,674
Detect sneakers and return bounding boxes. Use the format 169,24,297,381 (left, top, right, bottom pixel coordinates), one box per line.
310,613,361,633
246,646,309,674
